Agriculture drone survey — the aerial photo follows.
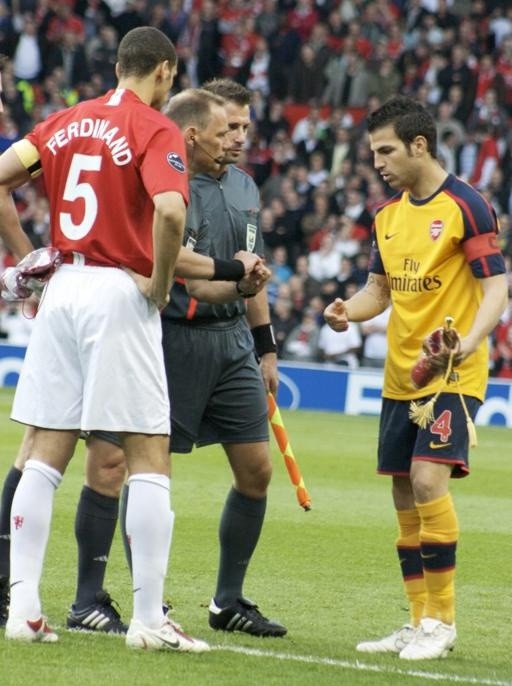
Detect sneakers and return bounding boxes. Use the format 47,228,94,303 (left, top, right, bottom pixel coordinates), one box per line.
205,600,288,638
400,618,457,660
7,613,59,645
65,602,130,633
356,624,417,655
123,620,212,656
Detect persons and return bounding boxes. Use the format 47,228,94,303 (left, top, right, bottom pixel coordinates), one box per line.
291,94,383,297
496,316,512,380
0,89,262,657
0,26,119,103
0,91,69,153
324,98,509,664
0,27,213,656
272,256,323,364
246,88,303,359
435,97,511,195
498,212,510,243
1,0,158,25
358,304,396,364
119,78,288,639
